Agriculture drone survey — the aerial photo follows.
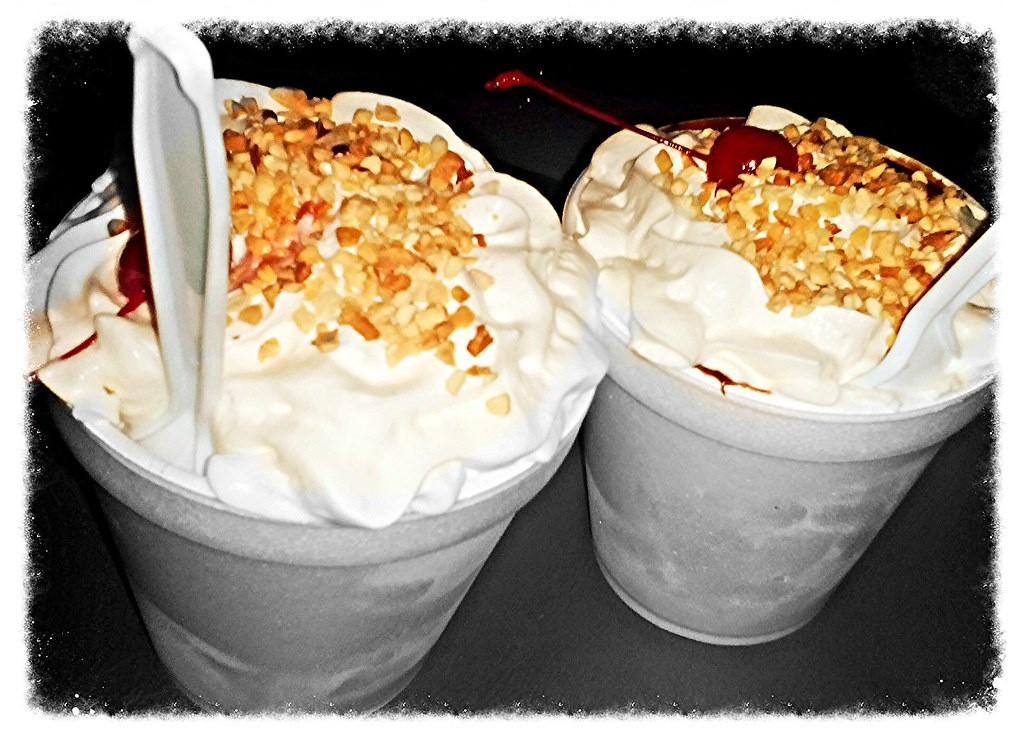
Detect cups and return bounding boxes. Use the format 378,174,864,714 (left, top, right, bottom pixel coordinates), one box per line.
558,112,998,648
33,243,597,716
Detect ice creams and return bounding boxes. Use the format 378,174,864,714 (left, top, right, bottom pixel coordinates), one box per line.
30,77,994,529
